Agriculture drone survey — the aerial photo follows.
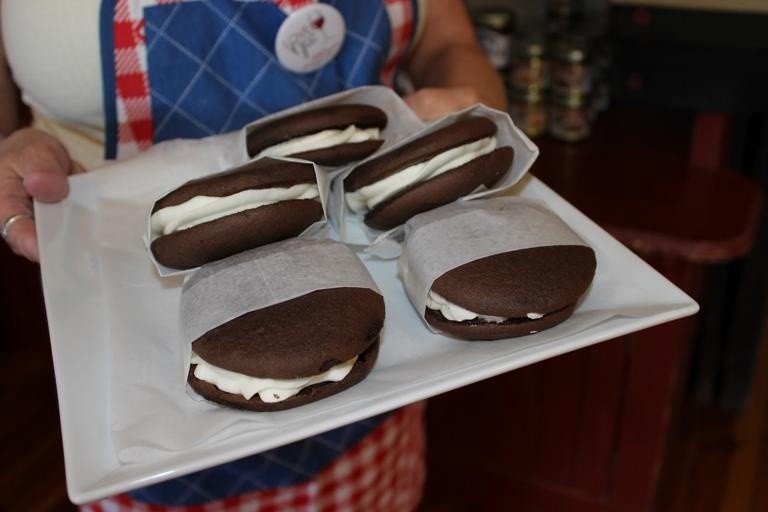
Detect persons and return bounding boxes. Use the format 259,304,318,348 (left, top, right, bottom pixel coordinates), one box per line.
0,1,511,512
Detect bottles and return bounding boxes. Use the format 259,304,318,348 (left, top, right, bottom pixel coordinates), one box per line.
477,10,607,140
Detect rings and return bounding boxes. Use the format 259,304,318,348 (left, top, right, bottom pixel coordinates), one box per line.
2,211,35,244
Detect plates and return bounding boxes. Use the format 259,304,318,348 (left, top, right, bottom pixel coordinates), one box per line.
33,128,700,505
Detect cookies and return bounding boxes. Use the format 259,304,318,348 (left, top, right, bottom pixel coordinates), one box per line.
189,288,386,410
425,244,597,342
246,103,388,167
343,115,515,231
151,164,324,270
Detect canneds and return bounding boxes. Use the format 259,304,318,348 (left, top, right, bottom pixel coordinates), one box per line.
472,11,598,143
